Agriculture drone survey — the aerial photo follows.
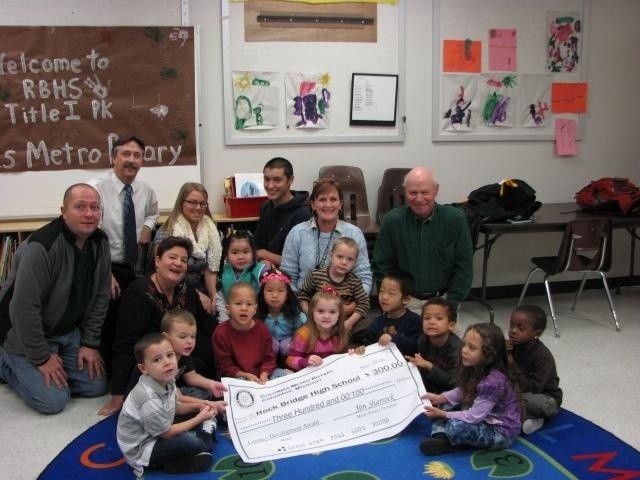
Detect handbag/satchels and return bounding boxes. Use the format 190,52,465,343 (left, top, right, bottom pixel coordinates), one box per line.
467,178,542,222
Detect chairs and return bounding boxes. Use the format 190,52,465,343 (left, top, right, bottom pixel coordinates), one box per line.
319,165,381,261
376,167,412,226
517,218,622,338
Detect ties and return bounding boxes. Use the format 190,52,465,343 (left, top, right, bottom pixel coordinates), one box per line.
123,184,138,266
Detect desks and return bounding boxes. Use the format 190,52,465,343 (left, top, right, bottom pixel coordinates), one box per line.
467,202,640,324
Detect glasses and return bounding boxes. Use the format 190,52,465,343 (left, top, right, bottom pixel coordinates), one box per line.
183,199,208,208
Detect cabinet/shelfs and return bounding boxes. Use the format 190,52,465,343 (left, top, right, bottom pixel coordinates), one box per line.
1,214,262,248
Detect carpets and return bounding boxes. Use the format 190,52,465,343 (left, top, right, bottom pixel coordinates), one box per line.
36,406,640,479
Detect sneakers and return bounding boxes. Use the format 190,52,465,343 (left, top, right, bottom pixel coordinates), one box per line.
418,432,449,456
198,417,217,436
165,451,213,473
522,416,544,435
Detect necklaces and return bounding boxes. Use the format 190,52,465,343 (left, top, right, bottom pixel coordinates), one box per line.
155,272,168,299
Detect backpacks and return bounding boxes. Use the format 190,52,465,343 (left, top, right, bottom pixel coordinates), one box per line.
461,179,542,249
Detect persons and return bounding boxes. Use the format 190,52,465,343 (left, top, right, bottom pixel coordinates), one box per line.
255,156,312,267
256,269,307,355
294,237,372,335
371,166,474,314
211,282,294,385
404,298,462,394
122,309,228,412
98,236,215,417
350,270,423,357
84,136,160,299
503,306,563,435
155,183,222,314
419,323,522,456
284,288,354,370
215,228,267,326
0,183,112,414
279,179,373,295
116,333,218,472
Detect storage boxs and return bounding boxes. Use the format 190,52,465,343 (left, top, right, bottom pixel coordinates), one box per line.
223,195,268,218
